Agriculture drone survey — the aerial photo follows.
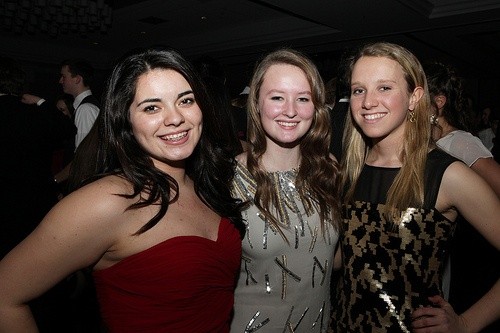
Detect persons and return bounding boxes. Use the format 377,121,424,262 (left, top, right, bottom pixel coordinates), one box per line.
0,58,108,260
333,42,499,333
417,57,499,201
223,48,345,333
0,50,244,333
